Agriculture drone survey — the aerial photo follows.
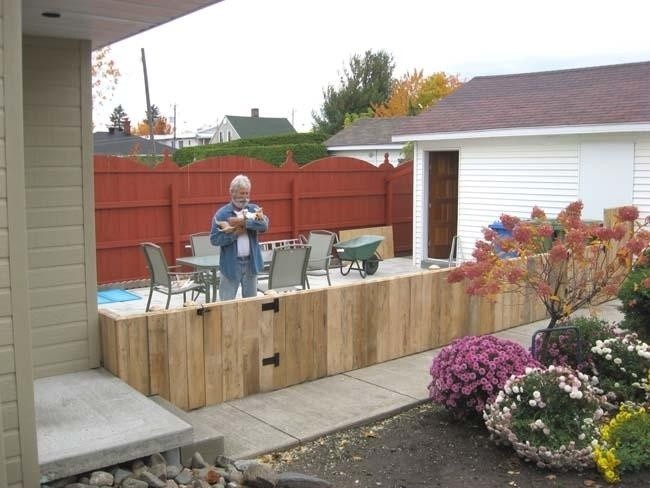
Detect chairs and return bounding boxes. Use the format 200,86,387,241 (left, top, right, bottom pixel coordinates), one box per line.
139,226,339,310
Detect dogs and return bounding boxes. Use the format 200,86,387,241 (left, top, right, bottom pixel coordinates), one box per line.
212,208,265,234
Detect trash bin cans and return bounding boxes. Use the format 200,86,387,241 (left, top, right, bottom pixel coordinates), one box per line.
489,221,525,260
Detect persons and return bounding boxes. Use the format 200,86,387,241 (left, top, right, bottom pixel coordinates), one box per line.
210,173,270,302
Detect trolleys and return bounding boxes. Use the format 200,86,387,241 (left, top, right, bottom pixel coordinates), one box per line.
334,235,385,278
